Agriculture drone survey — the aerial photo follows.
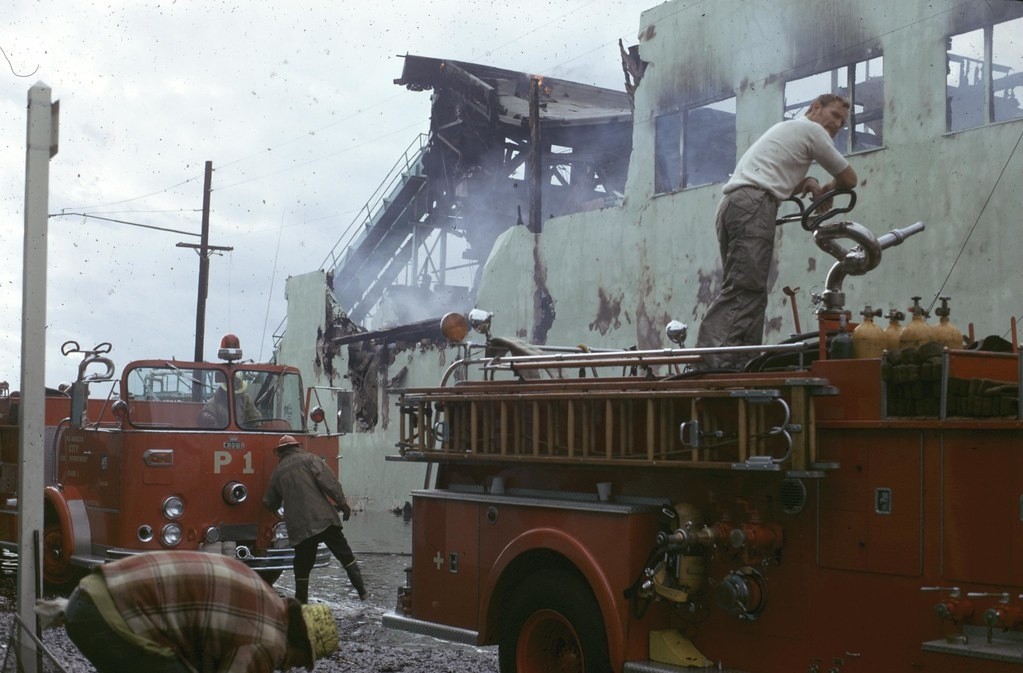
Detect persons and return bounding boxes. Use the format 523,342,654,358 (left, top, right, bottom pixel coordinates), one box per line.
64,551,340,673
263,435,371,605
691,94,857,369
201,371,262,429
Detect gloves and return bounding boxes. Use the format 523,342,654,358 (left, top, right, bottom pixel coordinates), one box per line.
343,509,350,522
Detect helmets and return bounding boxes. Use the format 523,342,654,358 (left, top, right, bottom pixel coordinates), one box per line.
300,602,338,673
220,372,247,394
277,435,301,448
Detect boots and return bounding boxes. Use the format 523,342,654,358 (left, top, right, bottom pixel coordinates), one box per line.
295,577,309,605
344,556,370,601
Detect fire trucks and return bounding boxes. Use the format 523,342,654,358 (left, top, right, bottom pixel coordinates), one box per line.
381,187,1023,673
0,334,341,597
